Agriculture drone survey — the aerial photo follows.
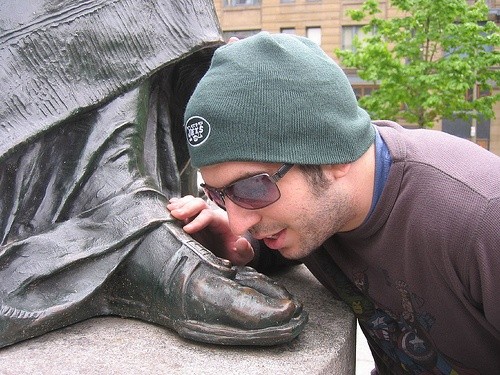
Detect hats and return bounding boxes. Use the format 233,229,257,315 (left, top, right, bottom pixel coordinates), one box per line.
184,31,376,169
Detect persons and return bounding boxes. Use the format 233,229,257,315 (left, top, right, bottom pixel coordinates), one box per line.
0,0,308,352
167,31,500,375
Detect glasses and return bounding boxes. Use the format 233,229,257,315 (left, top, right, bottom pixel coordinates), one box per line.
201,160,294,212
